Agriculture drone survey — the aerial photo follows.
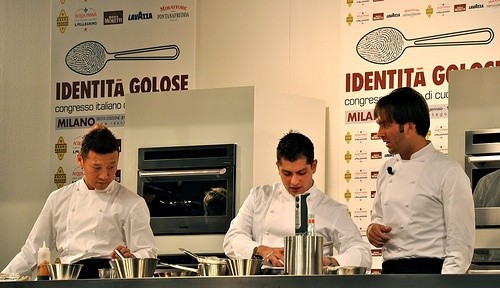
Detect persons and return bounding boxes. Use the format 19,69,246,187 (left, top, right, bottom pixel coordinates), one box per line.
204,187,233,231
0,123,158,278
223,133,372,270
472,169,500,262
366,87,475,274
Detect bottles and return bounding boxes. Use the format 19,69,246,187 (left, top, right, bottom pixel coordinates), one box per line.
307,217,316,236
37,240,51,280
253,252,263,274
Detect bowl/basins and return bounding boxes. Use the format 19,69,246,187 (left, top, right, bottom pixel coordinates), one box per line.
225,258,265,275
98,268,110,278
49,263,84,280
338,266,366,275
109,258,160,278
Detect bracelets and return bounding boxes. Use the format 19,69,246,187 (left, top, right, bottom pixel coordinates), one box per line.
328,258,332,266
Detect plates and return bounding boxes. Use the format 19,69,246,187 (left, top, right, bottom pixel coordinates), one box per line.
261,268,284,274
0,273,19,282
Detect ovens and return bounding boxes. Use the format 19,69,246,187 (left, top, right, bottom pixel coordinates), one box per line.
465,130,500,274
137,145,236,235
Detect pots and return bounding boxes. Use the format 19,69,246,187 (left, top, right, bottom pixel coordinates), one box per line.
179,248,228,276
284,235,323,275
